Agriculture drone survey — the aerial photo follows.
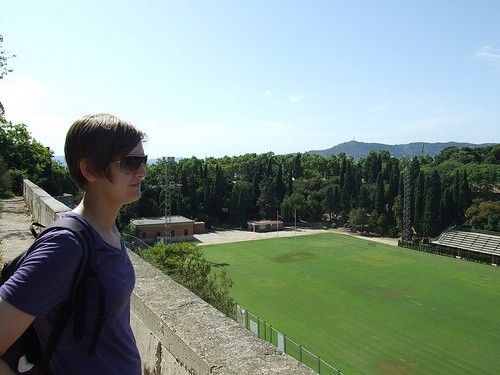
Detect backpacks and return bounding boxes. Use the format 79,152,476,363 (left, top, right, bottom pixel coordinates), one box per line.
0,217,95,374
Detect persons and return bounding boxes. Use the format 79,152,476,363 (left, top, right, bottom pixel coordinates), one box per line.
0,113,147,375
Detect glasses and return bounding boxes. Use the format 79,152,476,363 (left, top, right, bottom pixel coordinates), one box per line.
114,153,149,174
